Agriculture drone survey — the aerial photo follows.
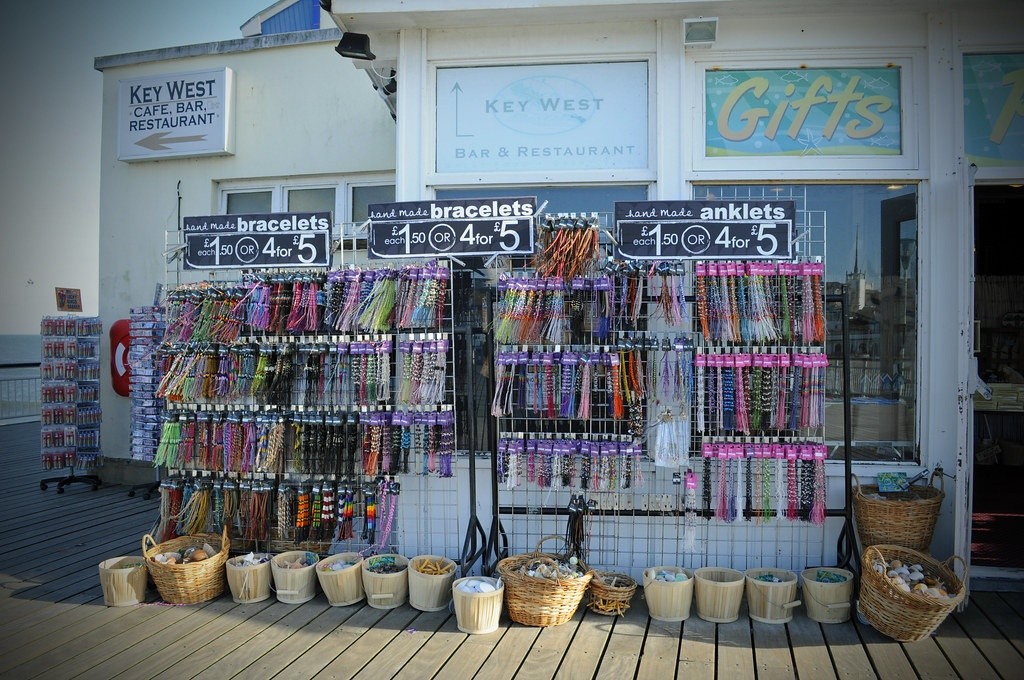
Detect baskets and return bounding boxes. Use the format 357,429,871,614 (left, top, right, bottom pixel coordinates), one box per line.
141,525,231,605
585,570,638,618
496,535,594,628
848,469,946,555
861,543,968,644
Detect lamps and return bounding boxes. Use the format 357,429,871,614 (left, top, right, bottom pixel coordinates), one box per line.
681,15,718,51
376,69,397,100
334,31,376,62
